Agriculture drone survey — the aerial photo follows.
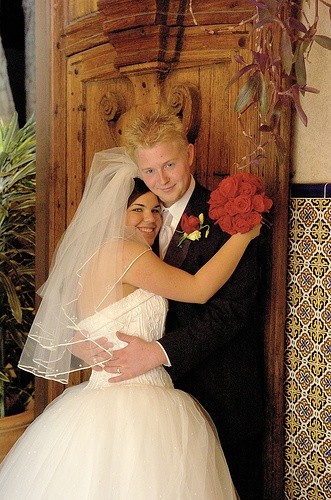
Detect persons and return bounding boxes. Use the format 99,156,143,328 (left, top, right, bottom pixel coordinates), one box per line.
67,108,259,500
0,147,263,499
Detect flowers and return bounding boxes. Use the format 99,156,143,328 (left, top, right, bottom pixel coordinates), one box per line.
176,213,210,248
207,173,272,235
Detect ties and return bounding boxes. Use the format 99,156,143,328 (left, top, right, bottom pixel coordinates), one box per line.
159,209,173,261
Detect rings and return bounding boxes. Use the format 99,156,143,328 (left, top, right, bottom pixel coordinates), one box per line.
117,367,121,373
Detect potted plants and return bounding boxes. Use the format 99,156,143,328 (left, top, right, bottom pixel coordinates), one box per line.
0,111,37,465
